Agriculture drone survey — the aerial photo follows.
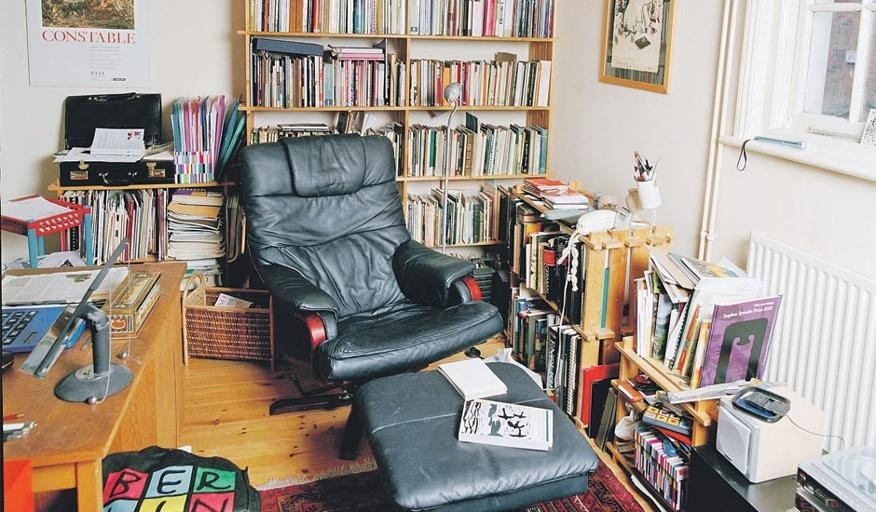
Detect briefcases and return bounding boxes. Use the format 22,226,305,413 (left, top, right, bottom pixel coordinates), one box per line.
60,160,177,187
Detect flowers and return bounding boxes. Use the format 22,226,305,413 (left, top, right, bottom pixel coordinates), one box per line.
632,153,653,180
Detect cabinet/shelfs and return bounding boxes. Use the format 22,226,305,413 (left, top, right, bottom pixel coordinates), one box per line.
237,29,554,247
509,191,670,430
606,334,730,512
46,182,238,295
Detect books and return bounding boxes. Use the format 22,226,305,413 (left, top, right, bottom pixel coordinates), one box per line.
247,1,402,178
408,1,554,246
438,188,779,511
1,94,246,268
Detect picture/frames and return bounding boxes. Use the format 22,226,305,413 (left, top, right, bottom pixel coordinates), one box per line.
596,0,675,96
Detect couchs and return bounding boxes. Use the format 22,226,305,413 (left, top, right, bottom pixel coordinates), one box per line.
240,134,503,461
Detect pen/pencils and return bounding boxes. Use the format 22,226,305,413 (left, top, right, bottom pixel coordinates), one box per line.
634,154,660,183
3,413,25,422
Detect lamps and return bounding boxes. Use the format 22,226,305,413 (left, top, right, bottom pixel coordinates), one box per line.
37,235,134,404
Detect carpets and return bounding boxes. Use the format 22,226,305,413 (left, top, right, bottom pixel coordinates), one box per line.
256,455,646,512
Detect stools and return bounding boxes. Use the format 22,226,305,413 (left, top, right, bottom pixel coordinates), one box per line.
340,361,599,510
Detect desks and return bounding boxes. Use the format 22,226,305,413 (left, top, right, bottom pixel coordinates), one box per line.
688,445,796,512
1,261,189,512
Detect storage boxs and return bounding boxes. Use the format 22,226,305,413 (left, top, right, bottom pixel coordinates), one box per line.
742,233,874,512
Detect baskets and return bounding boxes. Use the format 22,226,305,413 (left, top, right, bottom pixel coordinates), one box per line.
2,194,91,238
181,271,275,372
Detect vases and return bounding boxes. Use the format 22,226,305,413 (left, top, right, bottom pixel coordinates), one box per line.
626,178,665,214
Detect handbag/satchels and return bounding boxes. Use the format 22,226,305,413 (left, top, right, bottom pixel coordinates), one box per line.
65,92,162,151
